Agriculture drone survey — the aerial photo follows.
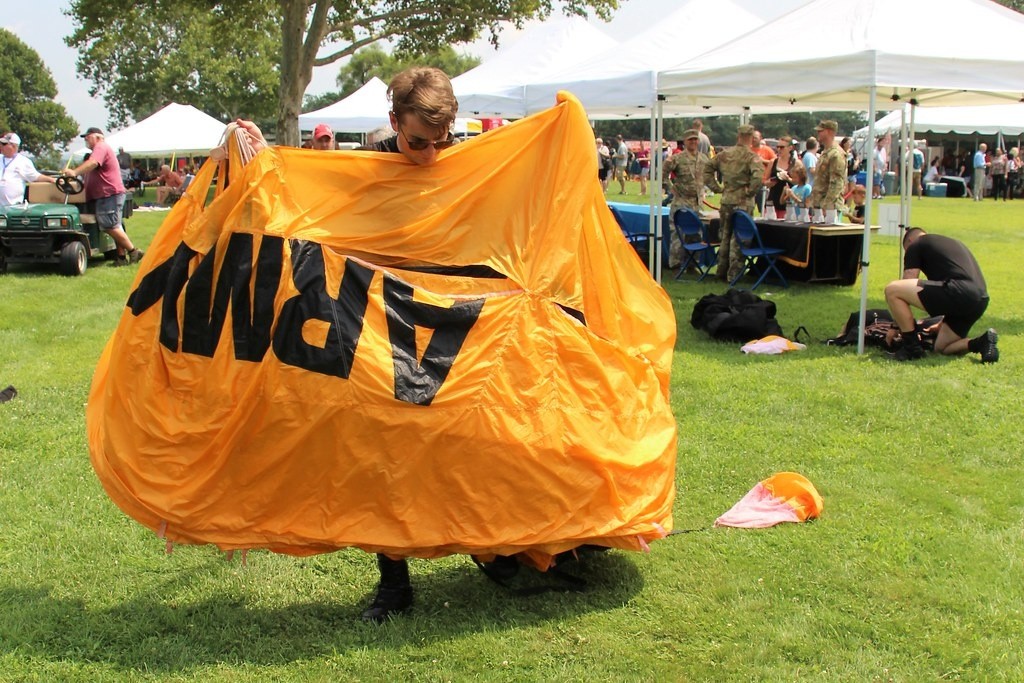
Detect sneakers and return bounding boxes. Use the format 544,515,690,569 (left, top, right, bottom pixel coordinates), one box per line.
128,248,144,264
106,257,128,267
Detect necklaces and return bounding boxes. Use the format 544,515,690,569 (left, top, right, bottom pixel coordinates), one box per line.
2,156,16,176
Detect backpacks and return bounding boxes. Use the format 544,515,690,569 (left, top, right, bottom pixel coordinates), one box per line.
914,153,922,169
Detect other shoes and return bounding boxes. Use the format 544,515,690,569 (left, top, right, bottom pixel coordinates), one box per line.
671,263,683,277
978,328,999,364
686,265,700,275
886,345,927,363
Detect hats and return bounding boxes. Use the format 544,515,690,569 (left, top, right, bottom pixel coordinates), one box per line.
315,124,332,140
684,130,698,139
792,140,799,145
80,127,103,137
0,133,21,146
738,125,754,133
814,120,838,132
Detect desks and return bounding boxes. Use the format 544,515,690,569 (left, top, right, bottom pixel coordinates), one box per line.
704,214,882,286
606,200,670,265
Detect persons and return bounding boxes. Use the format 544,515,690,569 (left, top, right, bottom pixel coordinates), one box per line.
117,147,201,208
870,137,1024,202
595,134,649,196
884,227,1000,364
662,117,856,283
0,133,63,214
310,124,333,150
65,127,144,266
228,67,459,615
366,125,393,145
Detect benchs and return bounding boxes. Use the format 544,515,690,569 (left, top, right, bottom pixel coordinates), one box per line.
28,181,99,248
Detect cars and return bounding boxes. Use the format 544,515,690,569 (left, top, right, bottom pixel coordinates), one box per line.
0,174,126,275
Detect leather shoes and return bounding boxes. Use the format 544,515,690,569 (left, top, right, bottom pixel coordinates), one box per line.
469,553,519,587
359,584,413,627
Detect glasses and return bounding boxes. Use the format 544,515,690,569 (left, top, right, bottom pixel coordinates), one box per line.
392,111,454,150
317,137,330,143
777,146,789,149
1,142,13,145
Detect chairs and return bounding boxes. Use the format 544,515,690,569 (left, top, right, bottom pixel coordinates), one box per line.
608,205,663,280
674,206,721,283
169,175,194,205
729,210,789,291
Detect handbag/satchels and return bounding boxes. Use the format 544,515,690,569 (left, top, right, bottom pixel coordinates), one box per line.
602,159,612,170
632,158,641,174
827,309,893,346
1008,170,1017,185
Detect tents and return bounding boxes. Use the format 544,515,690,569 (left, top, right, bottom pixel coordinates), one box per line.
852,101,1024,172
656,0,1024,353
77,102,227,158
298,76,510,152
445,17,624,141
525,0,767,277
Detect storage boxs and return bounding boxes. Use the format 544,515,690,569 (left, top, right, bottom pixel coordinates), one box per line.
925,182,948,196
